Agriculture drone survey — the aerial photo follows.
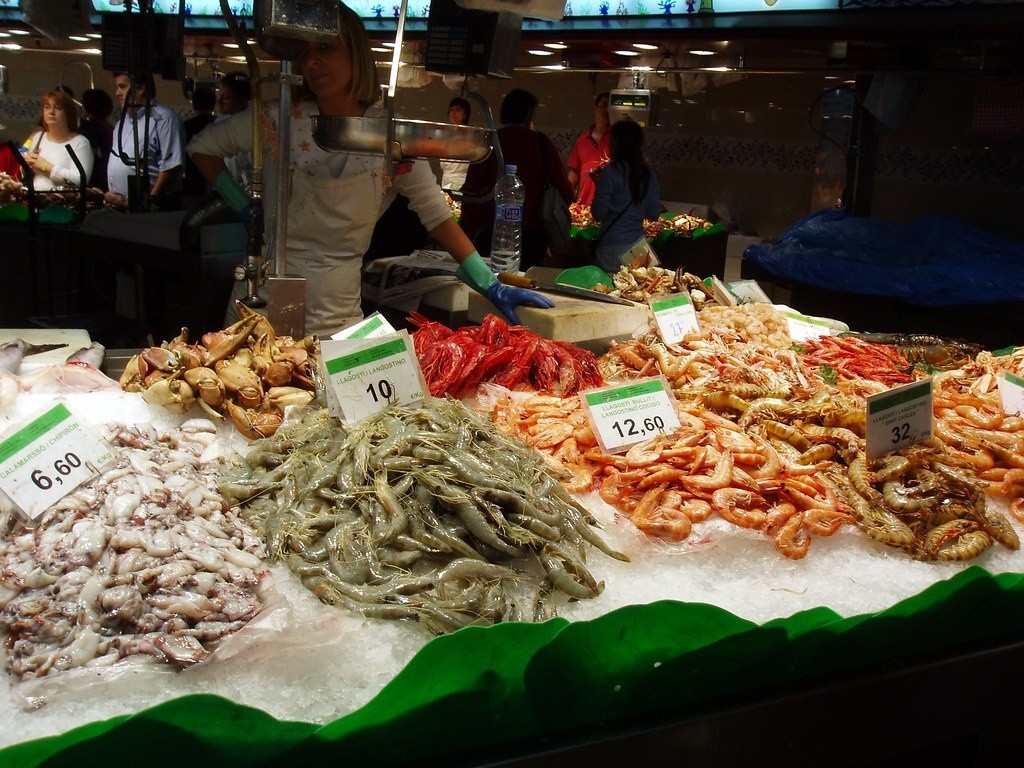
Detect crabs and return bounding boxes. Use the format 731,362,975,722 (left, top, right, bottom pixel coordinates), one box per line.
589,207,714,311
118,299,323,440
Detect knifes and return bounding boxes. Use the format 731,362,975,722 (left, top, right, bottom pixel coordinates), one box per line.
497,272,636,307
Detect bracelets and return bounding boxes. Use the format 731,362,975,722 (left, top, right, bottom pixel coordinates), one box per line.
41,162,49,174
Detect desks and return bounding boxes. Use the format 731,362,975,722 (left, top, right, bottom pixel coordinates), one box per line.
570,222,726,281
0,484,1024,767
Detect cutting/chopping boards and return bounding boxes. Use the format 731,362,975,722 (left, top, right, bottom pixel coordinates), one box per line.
463,284,650,344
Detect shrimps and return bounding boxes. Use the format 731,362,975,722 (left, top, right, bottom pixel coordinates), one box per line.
489,300,1024,562
407,308,602,401
213,390,631,639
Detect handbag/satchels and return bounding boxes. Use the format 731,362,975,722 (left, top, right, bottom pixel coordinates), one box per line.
539,183,571,256
589,240,597,260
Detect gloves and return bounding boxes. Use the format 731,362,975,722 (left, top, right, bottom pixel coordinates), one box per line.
455,250,555,326
212,168,264,232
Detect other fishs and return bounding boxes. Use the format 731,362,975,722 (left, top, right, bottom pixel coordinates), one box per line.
0,337,105,376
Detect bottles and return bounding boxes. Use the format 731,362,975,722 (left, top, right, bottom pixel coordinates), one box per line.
489,163,526,277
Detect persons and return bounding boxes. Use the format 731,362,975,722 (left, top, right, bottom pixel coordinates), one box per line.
0,70,254,210
185,2,550,339
440,88,661,274
